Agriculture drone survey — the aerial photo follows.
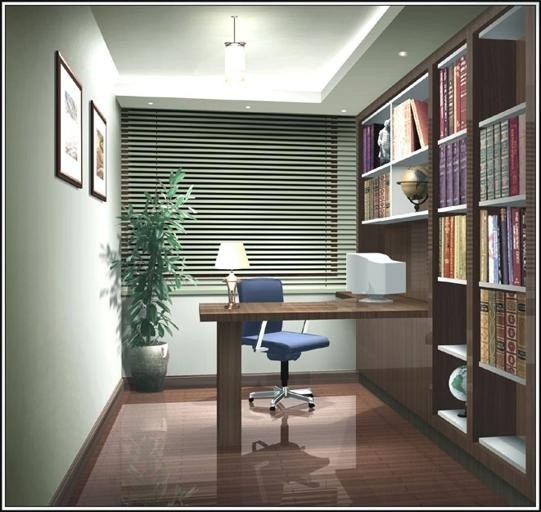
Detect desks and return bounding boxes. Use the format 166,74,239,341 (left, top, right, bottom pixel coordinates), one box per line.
199,292,429,454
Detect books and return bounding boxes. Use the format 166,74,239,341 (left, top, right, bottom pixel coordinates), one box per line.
361,124,392,220
479,113,527,380
437,55,468,281
392,98,428,160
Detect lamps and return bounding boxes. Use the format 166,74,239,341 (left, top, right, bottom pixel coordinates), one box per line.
225,16,246,83
215,241,250,309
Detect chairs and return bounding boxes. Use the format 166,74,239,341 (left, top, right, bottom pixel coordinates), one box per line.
238,279,329,411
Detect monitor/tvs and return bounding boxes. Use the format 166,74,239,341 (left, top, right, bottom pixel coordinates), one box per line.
347,251,407,305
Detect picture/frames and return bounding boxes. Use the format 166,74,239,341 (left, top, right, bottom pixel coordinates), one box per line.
91,100,107,202
55,51,83,188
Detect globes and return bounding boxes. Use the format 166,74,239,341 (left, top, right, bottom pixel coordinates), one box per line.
449,364,468,417
396,166,428,211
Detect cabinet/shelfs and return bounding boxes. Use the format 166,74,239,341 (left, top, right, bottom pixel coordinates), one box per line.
356,6,536,507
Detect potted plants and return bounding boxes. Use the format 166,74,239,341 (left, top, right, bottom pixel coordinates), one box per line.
110,167,196,392
